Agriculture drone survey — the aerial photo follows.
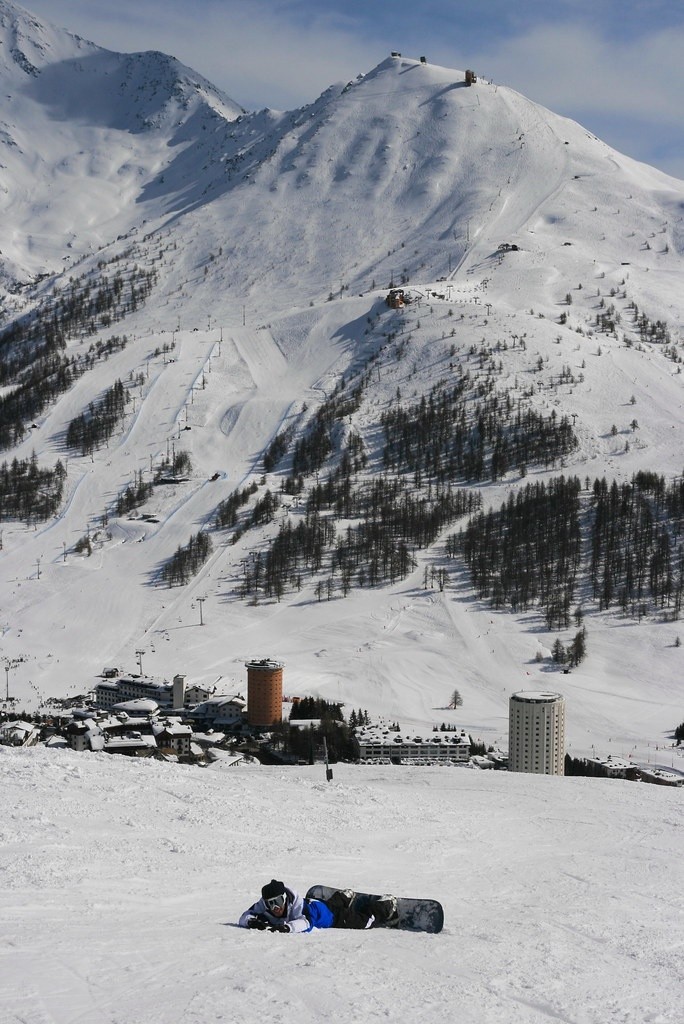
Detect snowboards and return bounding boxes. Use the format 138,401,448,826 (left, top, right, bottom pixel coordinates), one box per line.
305,884,444,934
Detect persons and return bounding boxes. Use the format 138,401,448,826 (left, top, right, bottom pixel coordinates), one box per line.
239,880,396,933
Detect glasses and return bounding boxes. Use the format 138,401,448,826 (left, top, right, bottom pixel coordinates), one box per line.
263,892,287,910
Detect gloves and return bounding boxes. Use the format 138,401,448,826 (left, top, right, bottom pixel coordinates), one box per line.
268,924,290,933
248,919,266,930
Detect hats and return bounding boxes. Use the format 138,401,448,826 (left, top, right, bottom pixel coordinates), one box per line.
261,880,284,899
